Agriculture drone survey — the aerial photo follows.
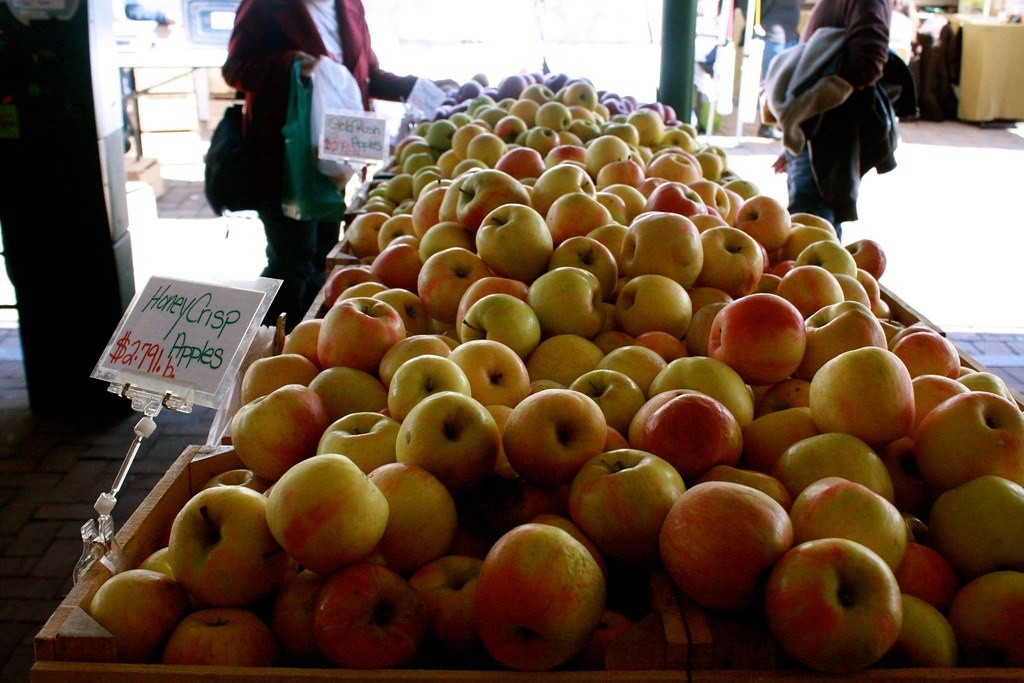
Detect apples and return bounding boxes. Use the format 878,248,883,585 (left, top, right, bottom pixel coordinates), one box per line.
86,73,1024,675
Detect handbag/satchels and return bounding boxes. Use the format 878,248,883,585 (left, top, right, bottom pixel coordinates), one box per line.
205,106,266,216
311,57,365,146
282,60,346,221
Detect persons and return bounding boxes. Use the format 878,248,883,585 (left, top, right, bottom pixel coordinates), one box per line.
759,0,801,140
219,0,460,335
772,0,891,243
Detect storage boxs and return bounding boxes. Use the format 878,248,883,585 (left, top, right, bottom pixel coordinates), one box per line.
125,157,165,200
